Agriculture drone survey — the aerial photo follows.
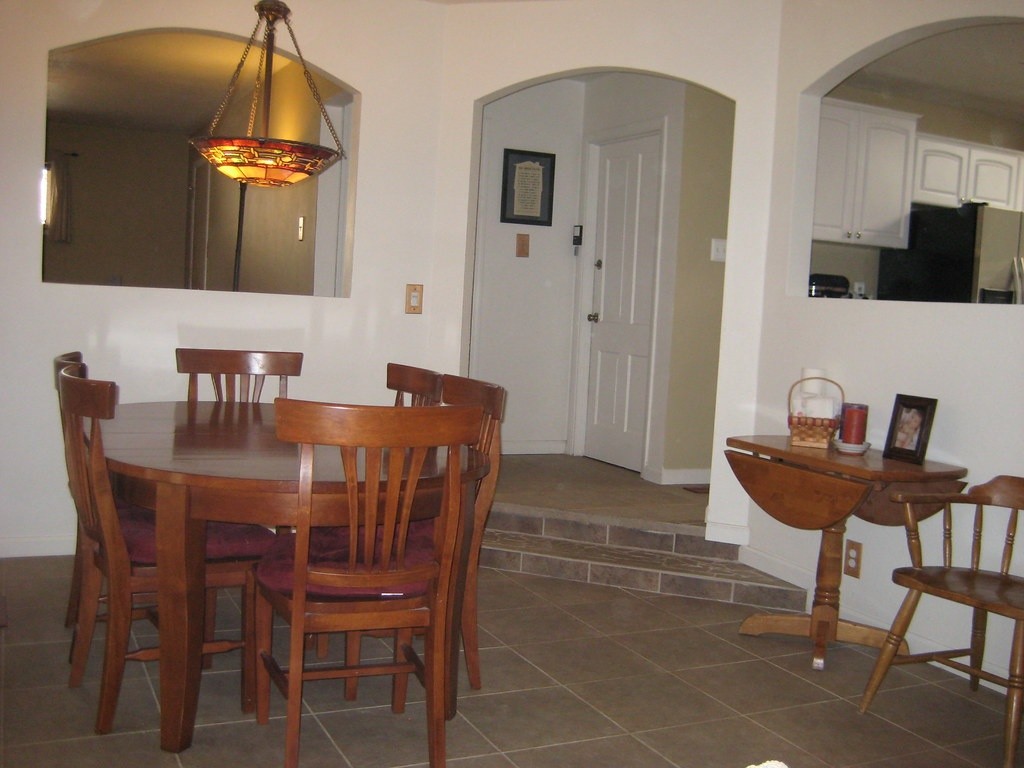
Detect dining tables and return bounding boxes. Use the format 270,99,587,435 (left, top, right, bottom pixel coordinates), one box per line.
80,399,491,756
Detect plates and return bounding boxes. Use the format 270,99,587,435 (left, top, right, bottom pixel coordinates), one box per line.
835,439,871,453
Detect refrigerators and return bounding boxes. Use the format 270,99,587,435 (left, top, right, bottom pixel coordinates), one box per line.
877,202,1024,304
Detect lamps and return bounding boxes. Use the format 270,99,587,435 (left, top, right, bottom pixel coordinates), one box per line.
188,0,345,188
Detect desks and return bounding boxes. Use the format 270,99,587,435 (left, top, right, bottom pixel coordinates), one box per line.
723,437,969,671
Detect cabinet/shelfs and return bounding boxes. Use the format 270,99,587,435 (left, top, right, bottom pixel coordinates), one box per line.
811,97,923,251
912,130,1024,218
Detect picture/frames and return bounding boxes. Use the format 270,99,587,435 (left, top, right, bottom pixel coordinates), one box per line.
881,394,938,467
500,148,556,228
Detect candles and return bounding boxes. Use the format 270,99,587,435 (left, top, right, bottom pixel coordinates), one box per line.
838,402,868,439
842,408,867,445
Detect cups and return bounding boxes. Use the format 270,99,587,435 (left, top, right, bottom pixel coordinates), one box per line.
840,403,867,442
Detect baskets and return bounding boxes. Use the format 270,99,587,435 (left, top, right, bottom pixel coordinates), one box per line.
787,377,846,449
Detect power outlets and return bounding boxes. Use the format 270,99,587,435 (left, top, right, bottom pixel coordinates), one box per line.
843,539,864,579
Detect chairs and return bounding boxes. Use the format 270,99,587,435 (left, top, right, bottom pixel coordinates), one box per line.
853,473,1024,768
52,345,507,768
